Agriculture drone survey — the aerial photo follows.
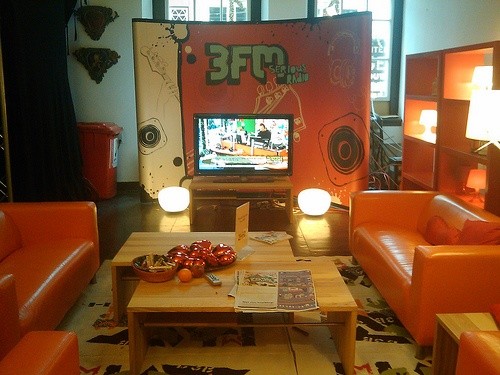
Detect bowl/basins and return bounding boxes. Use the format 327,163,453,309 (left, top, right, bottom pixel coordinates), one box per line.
131,254,178,282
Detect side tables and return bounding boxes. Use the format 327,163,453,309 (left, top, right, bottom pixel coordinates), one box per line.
431,312,500,375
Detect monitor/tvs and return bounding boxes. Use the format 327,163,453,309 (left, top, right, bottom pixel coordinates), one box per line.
193,112,294,183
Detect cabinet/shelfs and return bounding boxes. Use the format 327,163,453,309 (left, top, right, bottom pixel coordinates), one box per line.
400,41,500,217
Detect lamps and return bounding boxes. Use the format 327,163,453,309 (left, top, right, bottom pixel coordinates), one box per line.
466,169,487,203
158,176,193,213
466,89,500,148
298,188,349,216
471,66,493,89
418,109,437,141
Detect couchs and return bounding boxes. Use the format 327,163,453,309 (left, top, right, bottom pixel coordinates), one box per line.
348,190,500,375
0,202,101,375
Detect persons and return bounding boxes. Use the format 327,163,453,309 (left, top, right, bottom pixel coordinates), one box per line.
237,124,271,144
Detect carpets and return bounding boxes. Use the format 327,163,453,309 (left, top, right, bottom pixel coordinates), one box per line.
55,256,434,375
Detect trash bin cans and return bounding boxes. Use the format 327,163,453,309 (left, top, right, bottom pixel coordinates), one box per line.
76,120,124,200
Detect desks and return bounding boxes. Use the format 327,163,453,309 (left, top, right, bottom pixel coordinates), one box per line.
189,180,293,224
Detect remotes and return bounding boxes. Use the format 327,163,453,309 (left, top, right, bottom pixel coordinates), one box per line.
203,272,222,286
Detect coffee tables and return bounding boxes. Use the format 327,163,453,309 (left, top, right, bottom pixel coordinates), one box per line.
110,232,358,375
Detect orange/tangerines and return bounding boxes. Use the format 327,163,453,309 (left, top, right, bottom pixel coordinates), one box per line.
177,268,192,281
